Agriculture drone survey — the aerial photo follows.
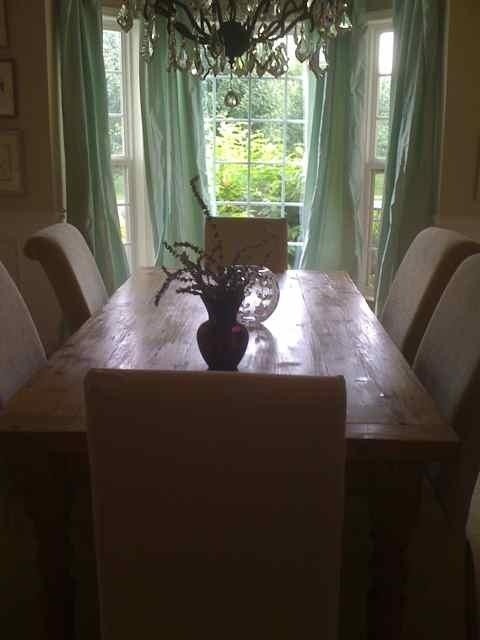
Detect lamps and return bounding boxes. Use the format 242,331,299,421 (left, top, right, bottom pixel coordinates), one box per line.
117,0,352,109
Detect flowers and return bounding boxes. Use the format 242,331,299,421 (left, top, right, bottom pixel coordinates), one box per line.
154,175,270,307
